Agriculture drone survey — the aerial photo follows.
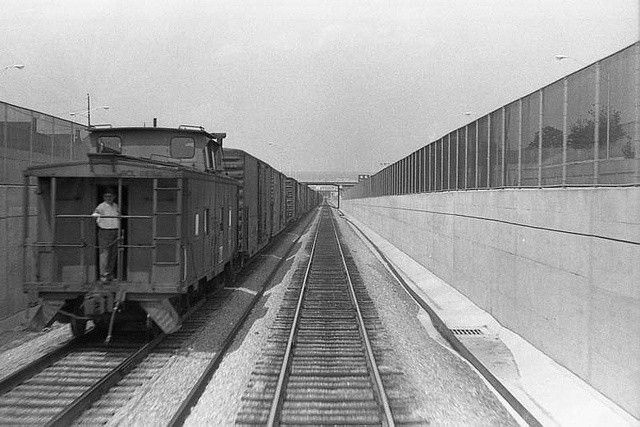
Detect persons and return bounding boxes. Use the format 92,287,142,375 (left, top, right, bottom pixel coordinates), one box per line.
90,186,125,285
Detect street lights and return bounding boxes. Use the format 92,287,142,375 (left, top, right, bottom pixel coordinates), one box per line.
1,61,25,83
556,55,609,160
66,103,113,127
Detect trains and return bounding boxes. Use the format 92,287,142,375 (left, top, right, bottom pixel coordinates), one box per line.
22,119,323,336
